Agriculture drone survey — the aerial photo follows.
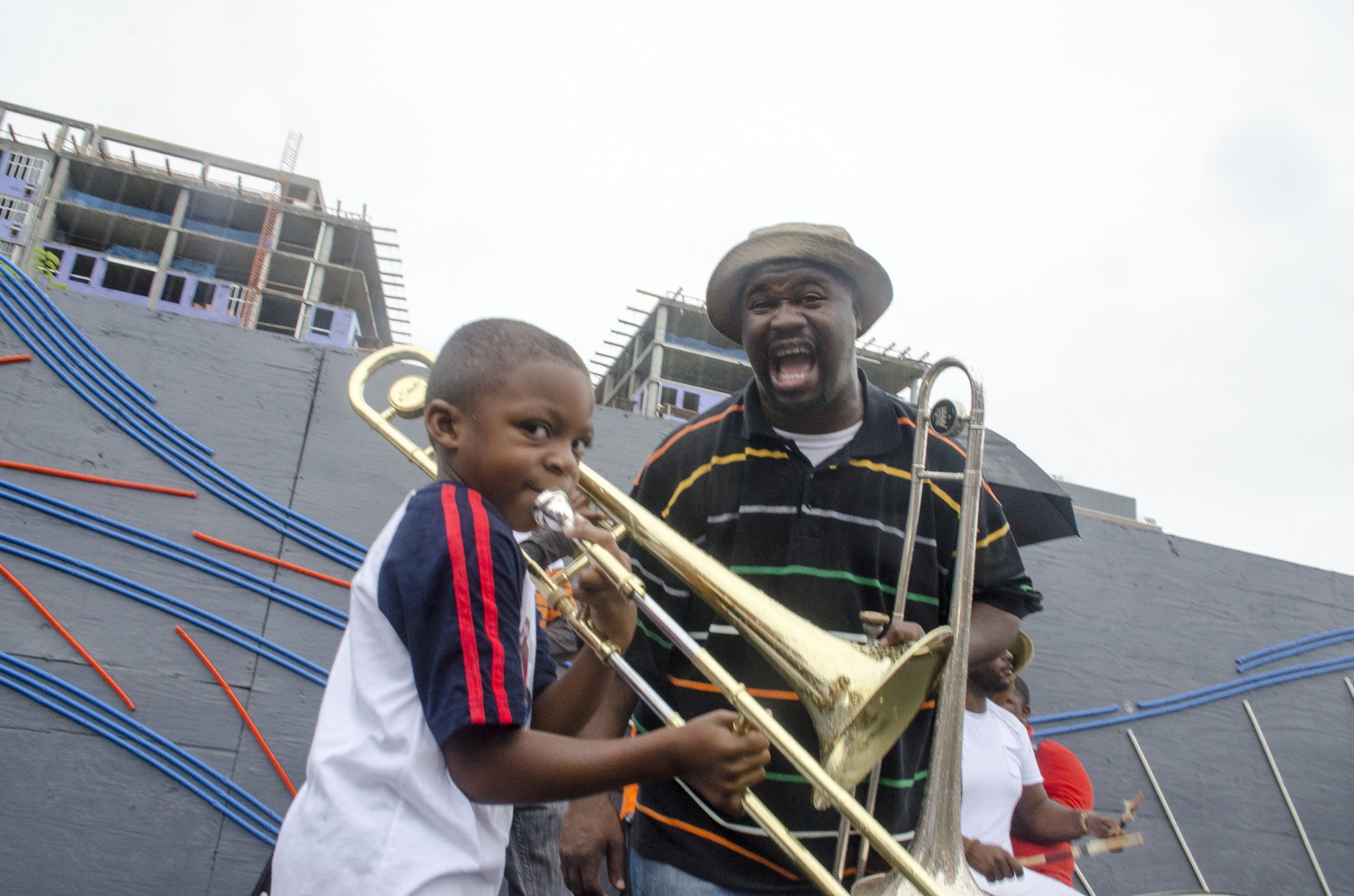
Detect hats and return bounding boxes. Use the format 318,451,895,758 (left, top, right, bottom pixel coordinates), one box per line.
705,221,892,344
1005,630,1033,676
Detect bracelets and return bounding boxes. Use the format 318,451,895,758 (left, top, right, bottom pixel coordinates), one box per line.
964,839,981,859
1080,810,1101,832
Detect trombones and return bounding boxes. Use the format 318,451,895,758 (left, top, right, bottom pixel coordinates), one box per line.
832,358,997,896
346,344,957,896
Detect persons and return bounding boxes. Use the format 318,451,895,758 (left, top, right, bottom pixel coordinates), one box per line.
1002,675,1094,888
556,223,1044,896
266,320,770,896
961,631,1128,896
